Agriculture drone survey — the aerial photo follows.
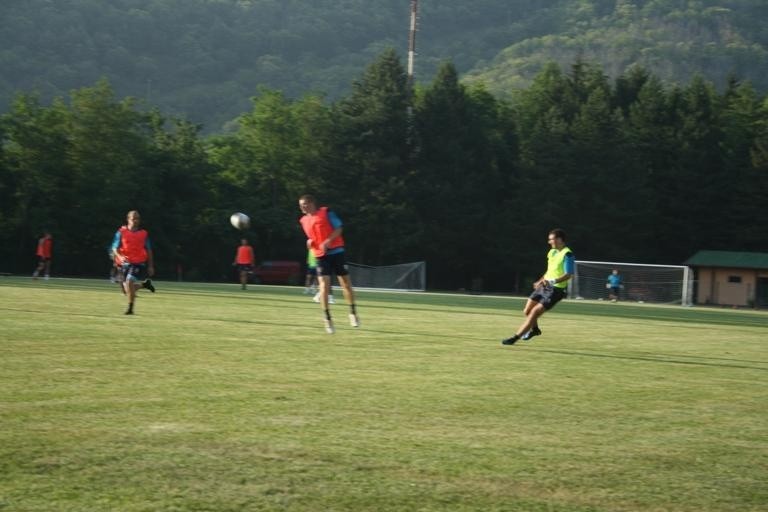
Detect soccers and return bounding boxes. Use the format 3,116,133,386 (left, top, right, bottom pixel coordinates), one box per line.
231,213,250,230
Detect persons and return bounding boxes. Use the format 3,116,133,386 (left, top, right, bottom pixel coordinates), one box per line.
502,229,575,344
32,230,57,280
108,210,155,314
233,239,256,289
303,239,317,294
606,269,624,302
298,194,359,334
313,263,336,304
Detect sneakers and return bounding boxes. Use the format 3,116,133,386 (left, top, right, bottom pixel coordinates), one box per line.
520,329,541,340
328,295,336,304
145,279,155,292
313,292,321,303
124,309,133,315
324,319,334,334
502,337,516,345
347,313,359,327
